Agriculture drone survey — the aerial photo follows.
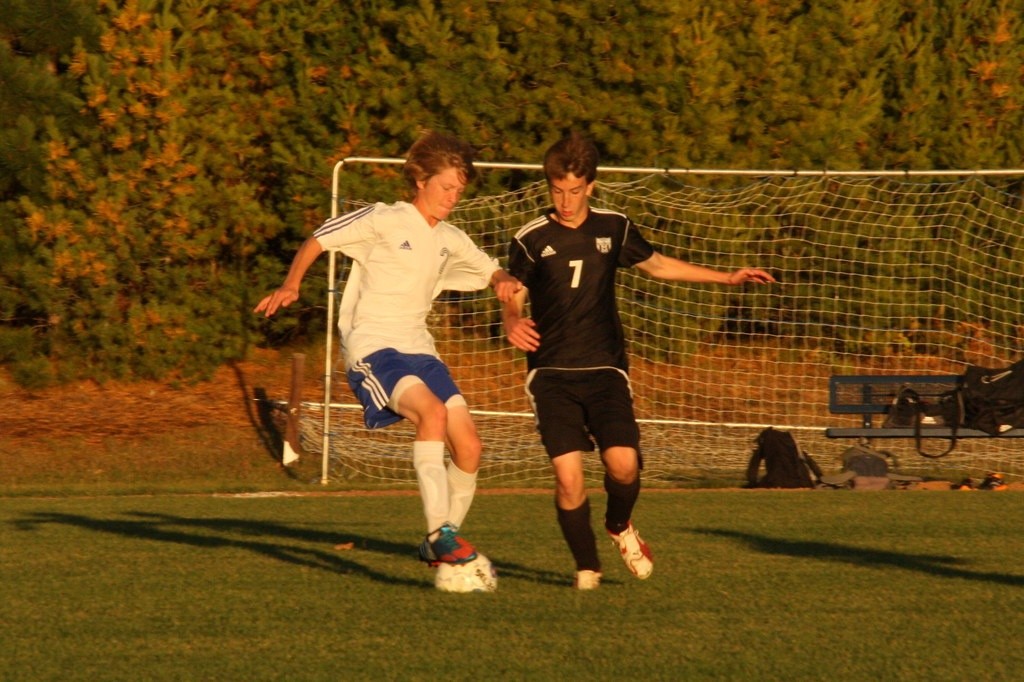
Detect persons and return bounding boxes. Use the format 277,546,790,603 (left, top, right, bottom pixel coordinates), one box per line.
508,140,775,592
255,132,525,563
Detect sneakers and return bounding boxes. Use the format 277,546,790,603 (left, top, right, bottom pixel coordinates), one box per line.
574,570,602,590
418,523,477,563
607,520,653,579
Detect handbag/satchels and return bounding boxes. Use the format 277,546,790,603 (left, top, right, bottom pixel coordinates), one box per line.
883,387,969,458
819,444,922,490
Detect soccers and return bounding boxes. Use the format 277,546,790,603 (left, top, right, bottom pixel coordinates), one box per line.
435,552,497,594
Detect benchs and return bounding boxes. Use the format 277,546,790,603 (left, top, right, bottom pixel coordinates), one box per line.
826,373,1024,442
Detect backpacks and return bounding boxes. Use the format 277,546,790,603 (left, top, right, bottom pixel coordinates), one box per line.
745,426,823,488
